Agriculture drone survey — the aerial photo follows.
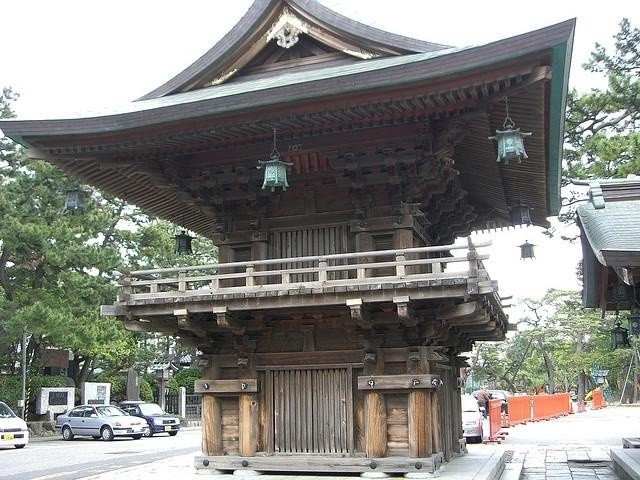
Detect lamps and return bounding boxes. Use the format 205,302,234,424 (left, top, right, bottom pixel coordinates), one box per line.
519,240,536,260
256,128,295,192
171,214,198,257
610,314,632,350
488,97,532,166
57,158,88,214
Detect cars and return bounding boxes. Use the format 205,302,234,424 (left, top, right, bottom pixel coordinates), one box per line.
56,404,149,441
0,402,29,448
461,394,484,442
118,402,180,437
472,390,515,413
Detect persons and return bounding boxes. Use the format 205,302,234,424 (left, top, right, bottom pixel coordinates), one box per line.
476,387,491,419
